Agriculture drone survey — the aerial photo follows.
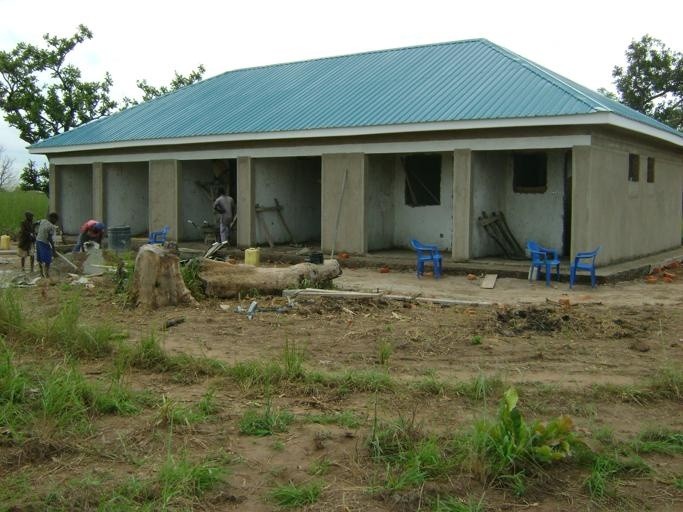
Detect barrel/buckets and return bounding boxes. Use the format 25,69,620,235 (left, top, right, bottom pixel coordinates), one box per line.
310,253,324,264
0,235,10,249
107,225,131,255
245,247,261,265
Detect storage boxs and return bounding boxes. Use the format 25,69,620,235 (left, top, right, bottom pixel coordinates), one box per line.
298,250,324,264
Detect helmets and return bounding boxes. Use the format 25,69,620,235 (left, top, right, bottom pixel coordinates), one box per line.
95,222,105,231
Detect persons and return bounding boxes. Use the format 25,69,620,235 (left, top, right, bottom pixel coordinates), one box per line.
30,212,59,279
15,210,37,273
69,219,105,254
212,187,235,244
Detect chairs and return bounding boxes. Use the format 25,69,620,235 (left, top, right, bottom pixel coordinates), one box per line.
526,239,560,287
148,225,170,246
569,246,599,289
410,239,443,279
